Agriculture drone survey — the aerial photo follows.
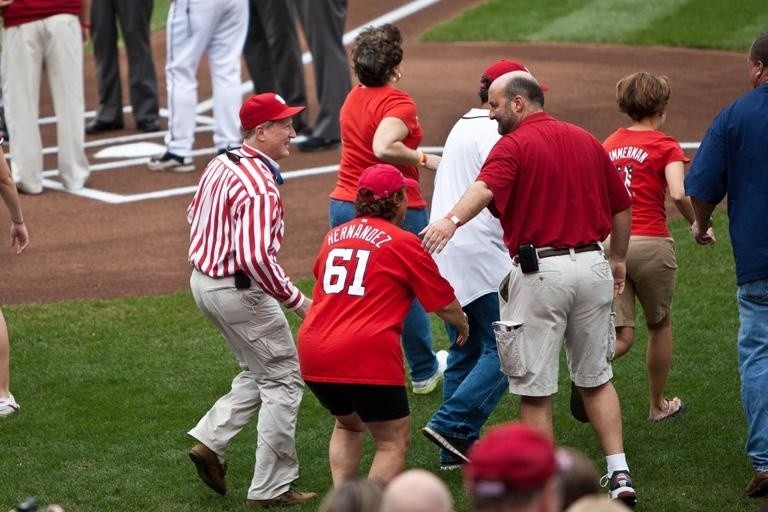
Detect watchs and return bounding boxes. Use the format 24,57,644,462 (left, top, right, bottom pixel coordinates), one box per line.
462,309,469,328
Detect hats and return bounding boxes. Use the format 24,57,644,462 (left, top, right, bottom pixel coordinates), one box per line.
238,94,305,128
481,58,550,92
462,423,557,490
358,163,418,200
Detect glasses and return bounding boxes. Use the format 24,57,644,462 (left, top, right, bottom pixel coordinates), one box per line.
225,145,260,165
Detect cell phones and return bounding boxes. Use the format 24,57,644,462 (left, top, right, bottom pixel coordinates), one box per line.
516,243,542,275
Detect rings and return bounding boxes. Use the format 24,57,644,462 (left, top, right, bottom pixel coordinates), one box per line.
467,333,470,338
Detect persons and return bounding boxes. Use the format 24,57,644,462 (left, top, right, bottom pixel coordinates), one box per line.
684,33,768,498
83,1,166,136
567,493,634,511
296,163,472,488
184,90,318,506
0,0,92,196
328,23,452,396
298,0,354,154
570,68,717,425
380,465,455,512
422,57,550,472
418,71,638,508
144,0,250,173
555,443,603,511
318,476,387,510
461,423,563,510
243,3,309,133
1,136,31,420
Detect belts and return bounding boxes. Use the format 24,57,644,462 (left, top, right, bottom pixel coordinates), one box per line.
515,244,602,262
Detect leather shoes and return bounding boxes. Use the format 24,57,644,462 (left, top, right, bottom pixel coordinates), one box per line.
301,133,340,153
85,117,127,133
136,118,162,131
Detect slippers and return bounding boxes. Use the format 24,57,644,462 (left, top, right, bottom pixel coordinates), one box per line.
647,397,684,423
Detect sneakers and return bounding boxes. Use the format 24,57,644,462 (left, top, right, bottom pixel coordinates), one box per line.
745,469,768,494
414,350,453,396
423,422,478,465
1,396,20,415
188,443,227,496
246,490,317,511
146,154,196,173
438,449,466,472
609,472,637,507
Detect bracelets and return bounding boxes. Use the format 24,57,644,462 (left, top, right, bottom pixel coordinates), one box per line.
445,210,463,228
417,152,428,170
10,218,24,225
